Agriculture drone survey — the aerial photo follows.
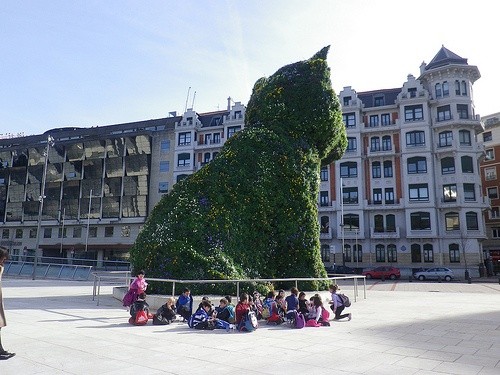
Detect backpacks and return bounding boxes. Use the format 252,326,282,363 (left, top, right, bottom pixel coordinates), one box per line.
134,310,148,326
122,290,137,306
153,313,170,324
315,306,330,322
244,308,258,331
334,293,351,307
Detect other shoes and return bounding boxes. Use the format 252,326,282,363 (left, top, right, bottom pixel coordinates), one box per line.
0,350,15,360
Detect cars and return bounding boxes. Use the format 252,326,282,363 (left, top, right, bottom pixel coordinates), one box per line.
414,266,455,282
324,266,401,280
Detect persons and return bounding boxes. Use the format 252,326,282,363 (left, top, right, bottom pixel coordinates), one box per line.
0,245,16,360
176,287,193,322
129,291,149,326
188,286,331,332
329,284,352,321
129,269,152,319
152,297,177,324
